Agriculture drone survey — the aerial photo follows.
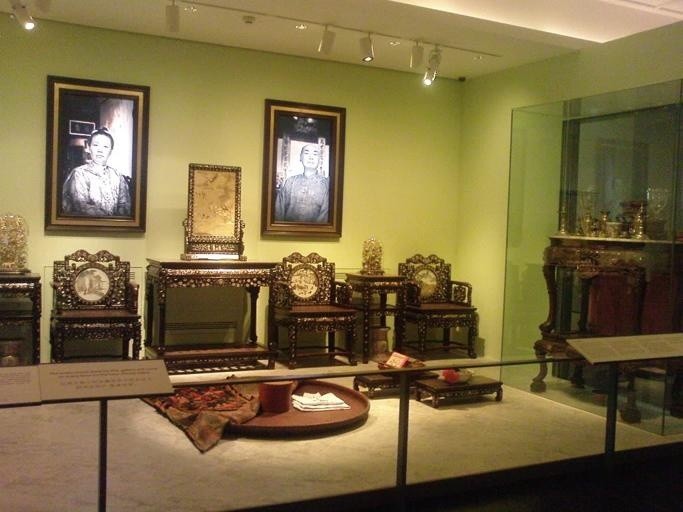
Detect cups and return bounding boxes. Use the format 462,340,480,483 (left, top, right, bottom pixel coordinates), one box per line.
255,381,300,410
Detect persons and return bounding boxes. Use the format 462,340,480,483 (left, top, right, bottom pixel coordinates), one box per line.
274,143,330,222
61,127,132,217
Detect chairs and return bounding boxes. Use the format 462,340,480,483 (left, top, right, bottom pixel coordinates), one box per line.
50,249,141,364
269,252,358,370
398,254,479,361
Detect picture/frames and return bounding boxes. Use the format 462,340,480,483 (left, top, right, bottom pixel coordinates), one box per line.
261,99,347,238
45,74,150,233
188,164,241,254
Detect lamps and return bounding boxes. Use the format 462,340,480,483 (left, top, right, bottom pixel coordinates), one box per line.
12,6,35,29
165,1,180,34
318,24,442,85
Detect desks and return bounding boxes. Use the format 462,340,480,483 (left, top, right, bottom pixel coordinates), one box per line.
145,258,284,369
354,371,503,408
345,273,408,364
530,235,683,423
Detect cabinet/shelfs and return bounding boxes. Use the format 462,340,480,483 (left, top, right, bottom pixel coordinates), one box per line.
0,273,41,366
